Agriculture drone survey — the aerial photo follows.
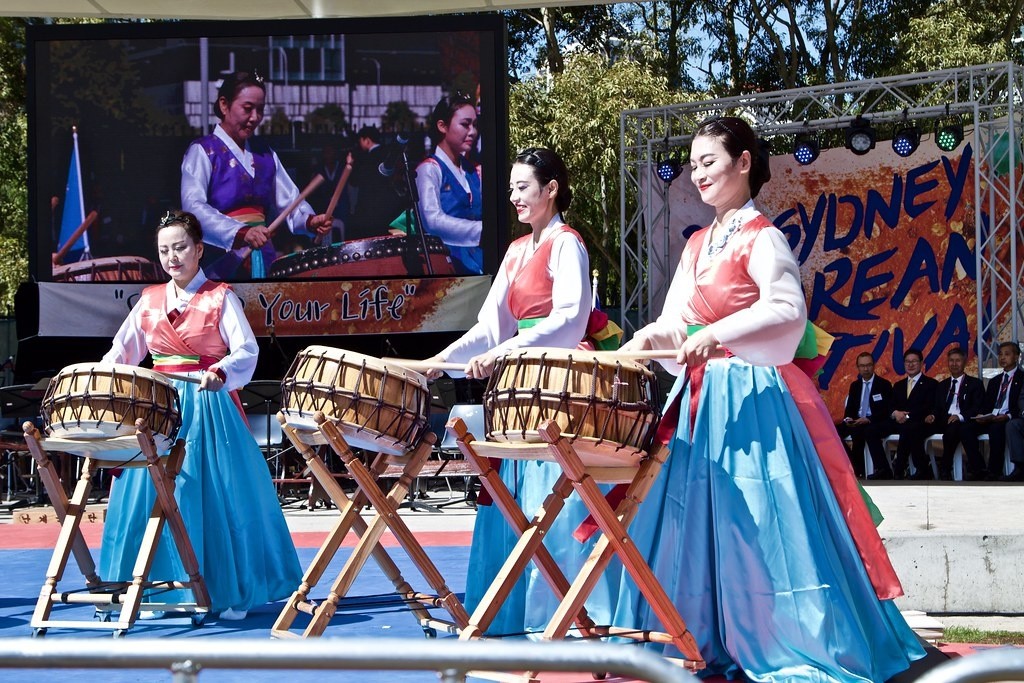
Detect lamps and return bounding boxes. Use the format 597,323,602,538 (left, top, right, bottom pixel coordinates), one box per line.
934,113,965,152
794,131,820,164
891,122,922,157
656,151,684,182
844,115,877,155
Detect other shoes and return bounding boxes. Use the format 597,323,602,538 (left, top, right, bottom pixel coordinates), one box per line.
867,469,894,480
939,469,953,481
985,470,1005,481
908,466,933,480
1005,467,1024,482
964,472,985,481
893,469,906,480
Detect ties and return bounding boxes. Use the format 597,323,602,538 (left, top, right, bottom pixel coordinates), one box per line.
861,381,870,418
906,378,915,398
945,380,957,412
994,373,1010,409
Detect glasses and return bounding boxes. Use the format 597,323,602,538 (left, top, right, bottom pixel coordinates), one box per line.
858,363,874,369
904,359,921,366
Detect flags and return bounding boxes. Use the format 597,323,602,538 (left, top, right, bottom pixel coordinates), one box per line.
56,144,85,265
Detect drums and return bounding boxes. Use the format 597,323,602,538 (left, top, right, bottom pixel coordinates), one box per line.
41,362,183,454
483,346,658,466
268,231,455,278
284,343,432,457
52,253,164,285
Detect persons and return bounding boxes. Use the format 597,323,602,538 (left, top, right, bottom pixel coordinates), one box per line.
420,147,623,648
866,349,940,481
181,71,334,281
311,92,484,275
835,352,893,479
98,213,303,621
908,348,985,482
607,117,927,683
959,341,1024,483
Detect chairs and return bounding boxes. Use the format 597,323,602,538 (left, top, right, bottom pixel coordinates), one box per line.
840,394,1012,483
